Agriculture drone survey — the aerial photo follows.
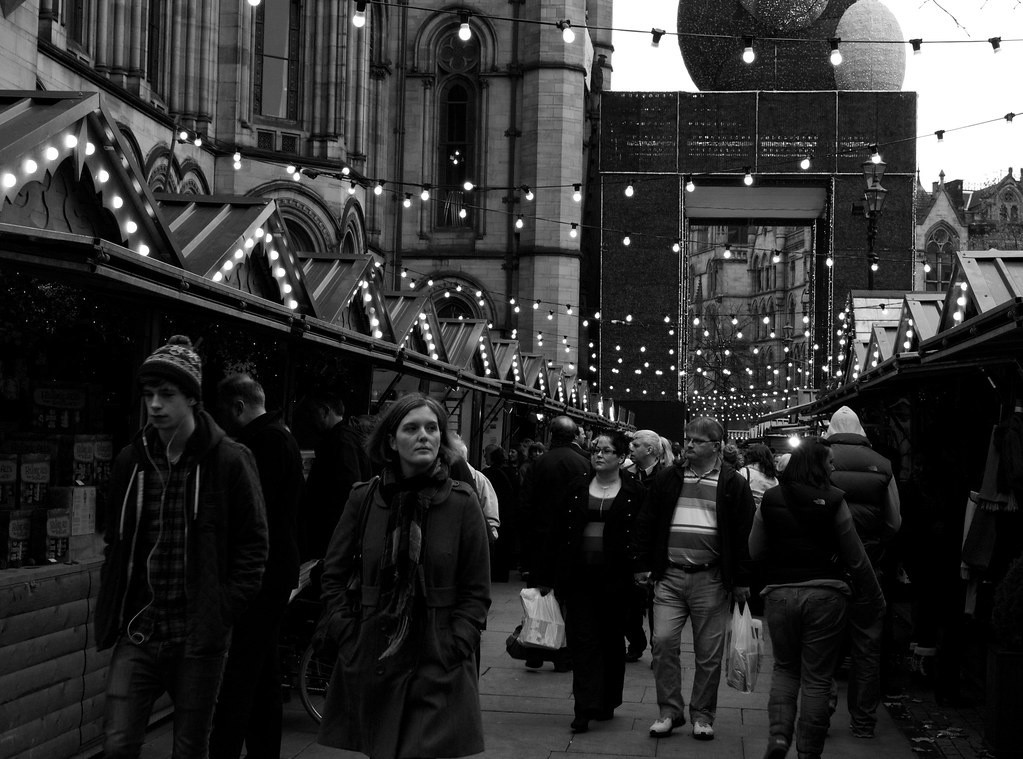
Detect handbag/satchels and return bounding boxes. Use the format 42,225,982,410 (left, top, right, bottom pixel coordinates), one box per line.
726,601,764,692
517,588,567,650
333,474,382,624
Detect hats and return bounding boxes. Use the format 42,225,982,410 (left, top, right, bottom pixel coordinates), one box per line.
138,336,202,401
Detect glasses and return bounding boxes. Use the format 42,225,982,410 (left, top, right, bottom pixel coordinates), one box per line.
594,448,623,455
683,437,718,444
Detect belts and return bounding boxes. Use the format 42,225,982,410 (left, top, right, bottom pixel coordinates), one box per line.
666,562,714,573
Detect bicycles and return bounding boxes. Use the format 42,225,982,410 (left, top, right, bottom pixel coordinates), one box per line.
279,620,329,727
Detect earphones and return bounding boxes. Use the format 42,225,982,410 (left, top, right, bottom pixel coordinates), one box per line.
190,397,195,404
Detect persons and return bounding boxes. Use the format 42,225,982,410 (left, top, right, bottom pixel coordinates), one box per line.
281,388,384,704
208,374,304,759
317,392,492,759
748,438,886,759
634,416,756,740
722,432,805,619
532,414,680,671
554,429,653,730
94,334,269,759
818,405,901,738
437,439,548,583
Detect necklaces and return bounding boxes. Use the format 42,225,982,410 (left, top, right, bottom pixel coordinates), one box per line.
596,476,620,510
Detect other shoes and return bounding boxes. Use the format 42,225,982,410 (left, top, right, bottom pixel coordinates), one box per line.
525,652,543,668
571,717,588,731
592,707,615,721
625,638,647,662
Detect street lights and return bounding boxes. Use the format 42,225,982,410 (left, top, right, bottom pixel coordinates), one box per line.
861,151,889,290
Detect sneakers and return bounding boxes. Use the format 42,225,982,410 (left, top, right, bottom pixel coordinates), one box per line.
694,721,714,740
650,714,687,736
